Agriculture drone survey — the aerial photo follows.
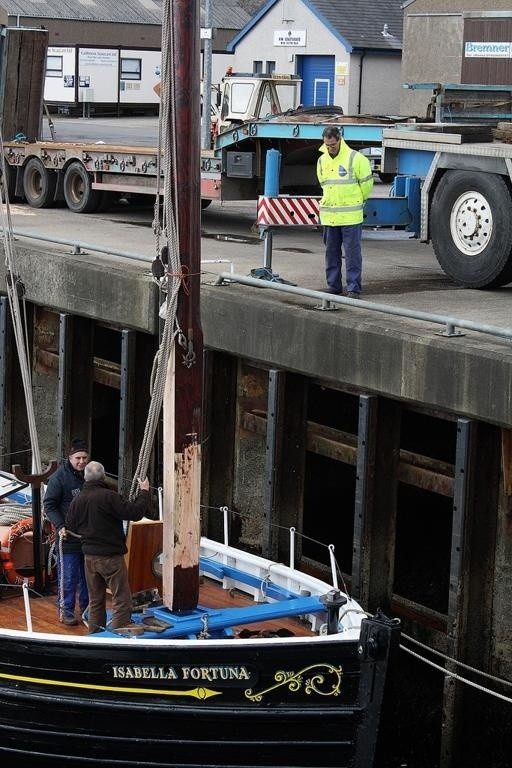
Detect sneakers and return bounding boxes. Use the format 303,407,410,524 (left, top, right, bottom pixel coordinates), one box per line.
62,617,77,625
347,292,359,298
114,621,143,629
322,287,342,293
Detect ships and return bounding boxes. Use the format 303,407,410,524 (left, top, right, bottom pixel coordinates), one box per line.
0,0,403,767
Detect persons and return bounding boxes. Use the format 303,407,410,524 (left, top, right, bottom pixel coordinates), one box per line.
44,437,89,625
65,461,150,635
315,127,375,301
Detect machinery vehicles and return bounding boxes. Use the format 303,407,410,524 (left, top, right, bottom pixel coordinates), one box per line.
1,82,511,287
151,71,301,147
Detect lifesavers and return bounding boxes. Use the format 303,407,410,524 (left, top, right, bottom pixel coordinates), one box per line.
1,518,61,589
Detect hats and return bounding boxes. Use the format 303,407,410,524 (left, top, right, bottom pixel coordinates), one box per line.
68,438,89,455
